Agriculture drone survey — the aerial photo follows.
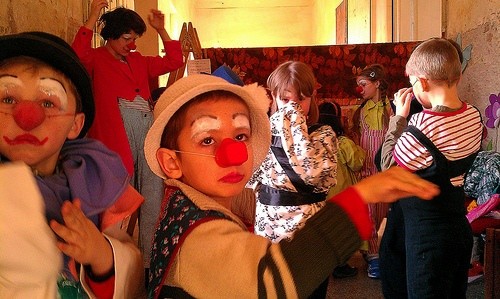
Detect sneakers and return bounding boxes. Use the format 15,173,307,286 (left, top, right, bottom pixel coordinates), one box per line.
368,258,382,278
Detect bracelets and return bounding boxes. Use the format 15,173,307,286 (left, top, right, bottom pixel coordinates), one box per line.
83,263,115,282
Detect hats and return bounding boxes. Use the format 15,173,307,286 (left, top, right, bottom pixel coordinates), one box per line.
0,31,96,140
143,73,272,182
212,66,243,85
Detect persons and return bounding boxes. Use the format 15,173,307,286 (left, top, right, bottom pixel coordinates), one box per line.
379,37,484,299
307,101,369,280
350,65,396,280
0,31,146,299
143,73,441,299
69,0,184,289
242,60,341,299
377,39,465,298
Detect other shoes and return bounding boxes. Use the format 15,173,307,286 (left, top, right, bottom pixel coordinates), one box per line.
468,260,484,283
332,263,358,278
